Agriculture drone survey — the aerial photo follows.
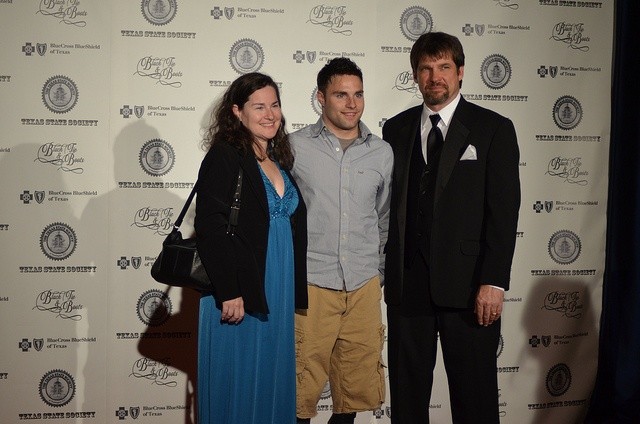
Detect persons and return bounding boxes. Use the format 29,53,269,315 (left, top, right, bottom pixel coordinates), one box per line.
382,32,520,424
281,57,394,424
195,72,307,424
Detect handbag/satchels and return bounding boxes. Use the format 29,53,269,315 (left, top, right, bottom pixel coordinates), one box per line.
150,228,243,291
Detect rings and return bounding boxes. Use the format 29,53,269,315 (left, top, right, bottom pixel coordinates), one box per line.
491,312,497,316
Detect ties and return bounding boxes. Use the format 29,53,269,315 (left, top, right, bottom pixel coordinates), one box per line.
427,114,443,170
